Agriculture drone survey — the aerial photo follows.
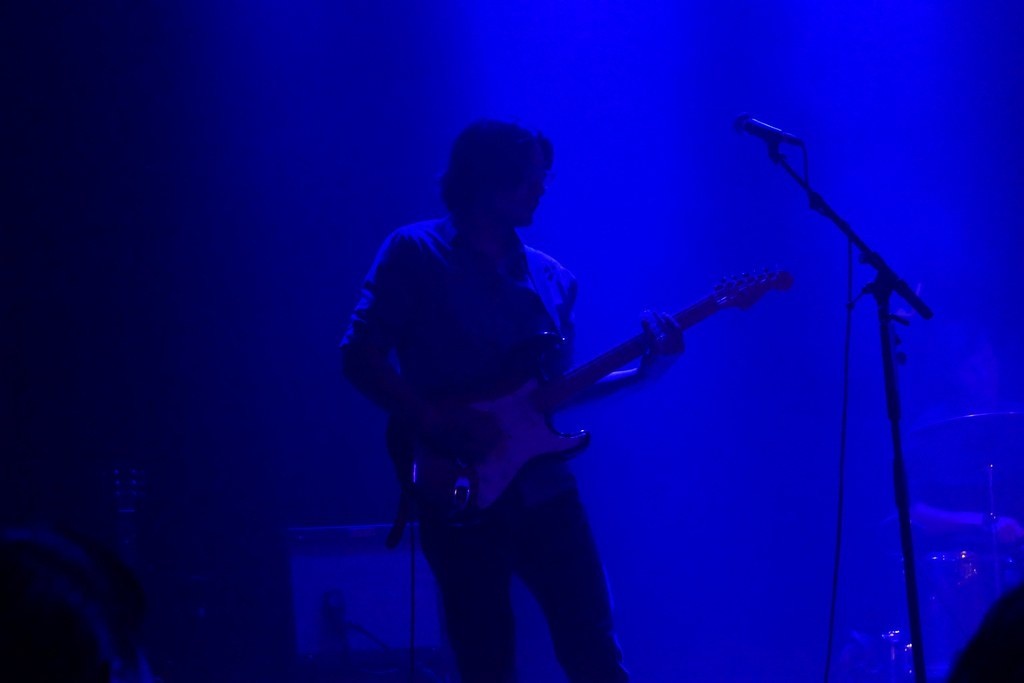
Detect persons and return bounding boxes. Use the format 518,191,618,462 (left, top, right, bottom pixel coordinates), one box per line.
344,109,686,682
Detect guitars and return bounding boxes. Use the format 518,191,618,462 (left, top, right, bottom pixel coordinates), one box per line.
406,269,796,524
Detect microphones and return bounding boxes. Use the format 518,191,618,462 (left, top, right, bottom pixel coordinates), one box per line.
735,113,809,150
